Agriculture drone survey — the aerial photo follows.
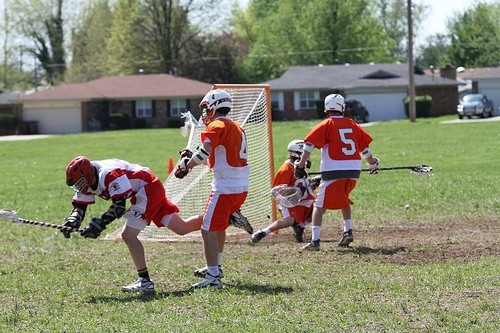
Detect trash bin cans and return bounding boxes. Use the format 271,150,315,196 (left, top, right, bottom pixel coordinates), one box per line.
20,121,38,135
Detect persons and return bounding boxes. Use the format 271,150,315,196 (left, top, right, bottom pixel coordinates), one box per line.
174,89,251,289
60,155,253,292
252,140,318,243
297,94,379,251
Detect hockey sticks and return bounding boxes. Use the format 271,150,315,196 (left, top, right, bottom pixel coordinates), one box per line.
270,177,322,208
0,208,85,232
176,110,202,177
306,164,433,175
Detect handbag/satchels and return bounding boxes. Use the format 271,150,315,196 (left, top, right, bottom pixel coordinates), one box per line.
174,157,192,178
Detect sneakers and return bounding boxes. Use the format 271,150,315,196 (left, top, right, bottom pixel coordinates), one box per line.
122,277,154,292
339,229,353,246
189,273,222,290
301,239,320,251
250,229,266,243
230,210,253,233
193,265,224,278
291,225,305,242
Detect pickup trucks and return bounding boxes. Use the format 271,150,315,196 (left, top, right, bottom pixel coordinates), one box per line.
457,92,494,119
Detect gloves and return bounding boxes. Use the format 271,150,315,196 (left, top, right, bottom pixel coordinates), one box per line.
60,216,81,238
368,159,380,174
294,159,307,179
81,217,106,239
179,149,192,158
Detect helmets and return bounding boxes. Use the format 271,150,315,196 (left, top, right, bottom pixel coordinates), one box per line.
199,90,232,125
66,156,94,194
287,140,310,161
324,94,346,117
